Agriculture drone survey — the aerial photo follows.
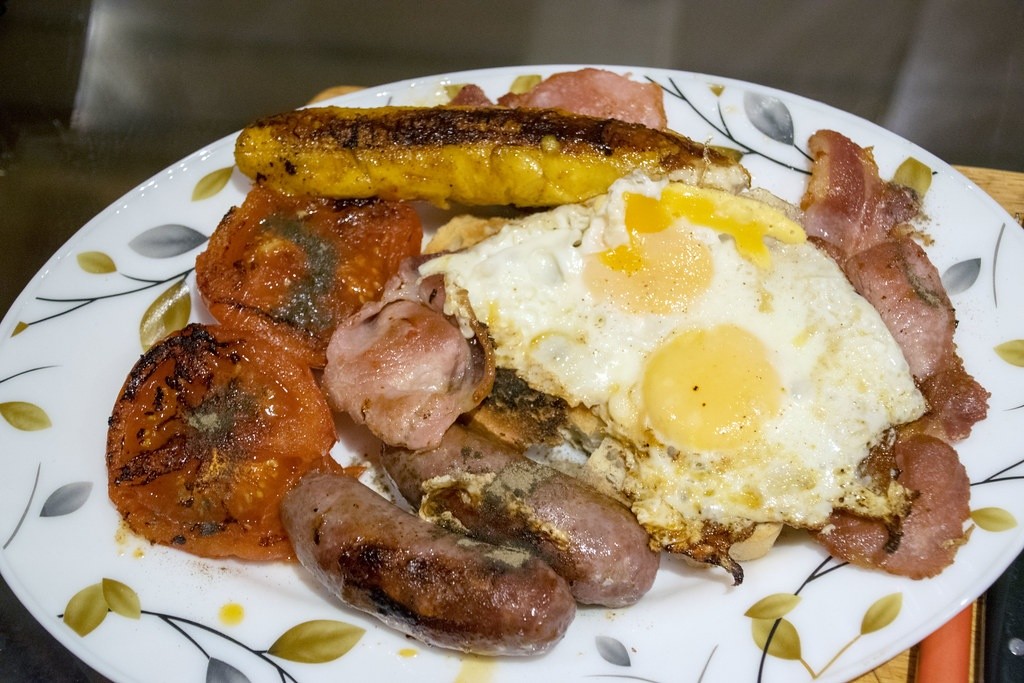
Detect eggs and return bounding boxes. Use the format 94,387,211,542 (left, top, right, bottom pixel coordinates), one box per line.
417,168,926,587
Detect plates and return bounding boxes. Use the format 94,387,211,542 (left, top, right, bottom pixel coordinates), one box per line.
0,65,1024,683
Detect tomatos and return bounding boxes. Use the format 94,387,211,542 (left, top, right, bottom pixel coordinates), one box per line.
107,187,423,564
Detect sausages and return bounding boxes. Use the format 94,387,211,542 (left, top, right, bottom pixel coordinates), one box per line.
280,434,662,658
